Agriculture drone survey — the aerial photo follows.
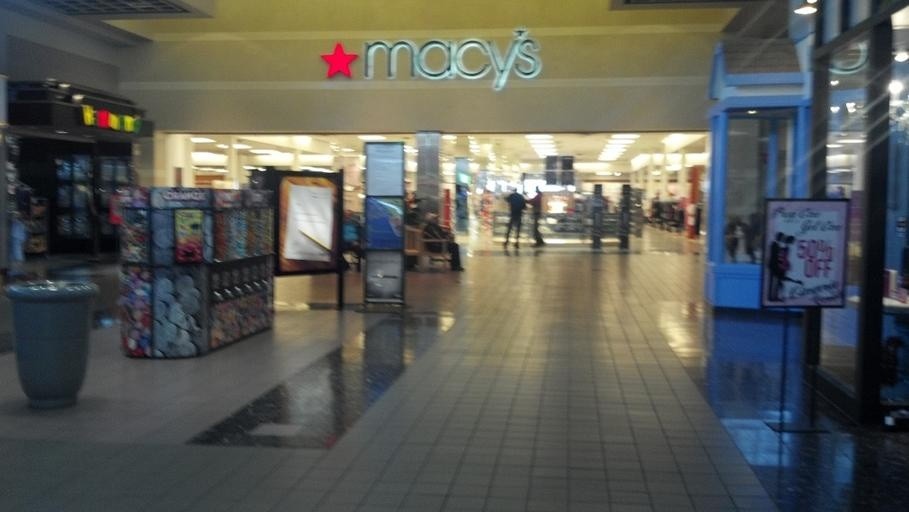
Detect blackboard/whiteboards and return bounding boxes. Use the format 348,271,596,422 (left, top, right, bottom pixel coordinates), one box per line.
268,168,344,273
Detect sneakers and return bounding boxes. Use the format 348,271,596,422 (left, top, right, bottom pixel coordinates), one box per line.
530,241,545,247
501,240,519,249
451,266,464,271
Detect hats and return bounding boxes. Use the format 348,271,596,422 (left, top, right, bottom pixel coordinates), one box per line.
423,212,441,222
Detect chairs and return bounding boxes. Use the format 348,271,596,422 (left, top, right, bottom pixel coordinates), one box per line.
403,223,459,274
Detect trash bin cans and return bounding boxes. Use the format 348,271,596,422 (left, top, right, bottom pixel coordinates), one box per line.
3,279,97,408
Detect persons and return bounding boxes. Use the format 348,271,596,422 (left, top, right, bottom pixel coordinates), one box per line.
525,186,546,247
502,187,528,250
772,234,804,301
336,209,365,270
767,232,784,301
424,212,466,272
404,191,422,272
725,223,762,264
